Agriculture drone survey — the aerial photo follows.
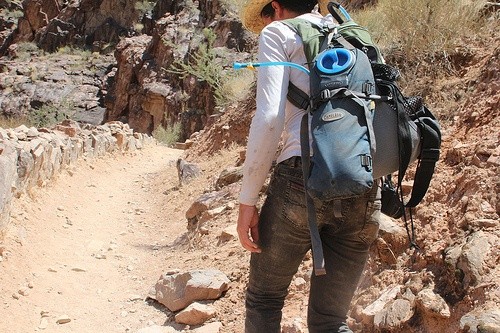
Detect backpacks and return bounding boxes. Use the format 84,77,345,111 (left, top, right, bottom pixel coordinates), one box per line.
278,4,442,211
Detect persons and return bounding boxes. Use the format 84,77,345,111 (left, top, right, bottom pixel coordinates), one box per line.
234,0,386,333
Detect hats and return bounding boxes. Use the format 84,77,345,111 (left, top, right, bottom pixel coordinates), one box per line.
239,1,332,33
